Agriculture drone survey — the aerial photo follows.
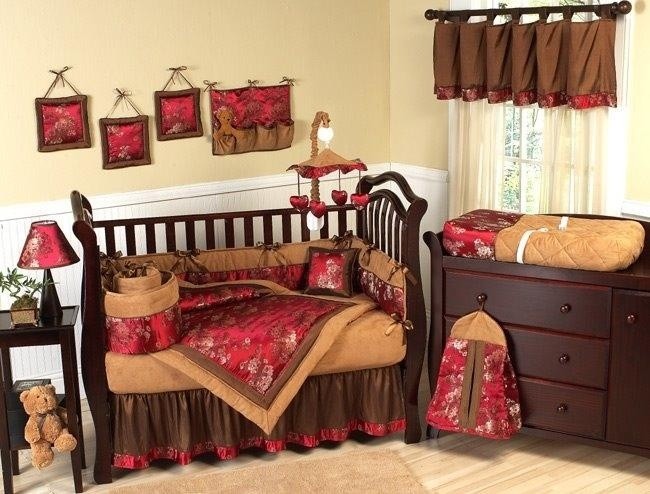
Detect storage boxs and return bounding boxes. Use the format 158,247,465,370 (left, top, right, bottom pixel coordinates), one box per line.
7,378,52,453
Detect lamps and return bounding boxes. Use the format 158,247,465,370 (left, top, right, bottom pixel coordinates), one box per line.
312,108,337,145
15,217,83,324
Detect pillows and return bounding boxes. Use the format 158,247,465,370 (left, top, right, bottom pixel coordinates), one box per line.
301,245,363,299
442,207,523,263
495,214,646,274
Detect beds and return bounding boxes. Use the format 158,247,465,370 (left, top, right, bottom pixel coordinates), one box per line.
63,166,436,487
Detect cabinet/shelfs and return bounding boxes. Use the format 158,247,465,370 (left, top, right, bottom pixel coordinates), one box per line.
421,209,650,458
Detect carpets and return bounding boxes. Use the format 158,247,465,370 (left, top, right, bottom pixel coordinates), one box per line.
110,446,431,494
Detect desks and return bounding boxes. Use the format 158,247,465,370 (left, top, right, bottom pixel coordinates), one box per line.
0,305,88,494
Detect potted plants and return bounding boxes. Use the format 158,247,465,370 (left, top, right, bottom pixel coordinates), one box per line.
0,270,59,328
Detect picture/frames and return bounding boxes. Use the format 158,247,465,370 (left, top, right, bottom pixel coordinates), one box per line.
98,113,152,170
153,85,203,143
33,92,92,153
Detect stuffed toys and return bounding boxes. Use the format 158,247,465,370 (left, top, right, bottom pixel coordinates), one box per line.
19,385,79,469
214,106,243,140
